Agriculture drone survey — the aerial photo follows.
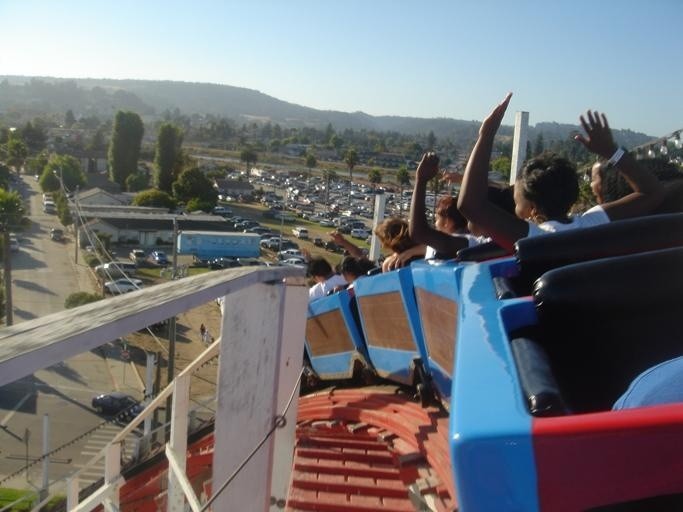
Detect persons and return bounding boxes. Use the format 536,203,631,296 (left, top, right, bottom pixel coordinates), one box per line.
585,151,678,204
299,152,519,308
458,92,661,239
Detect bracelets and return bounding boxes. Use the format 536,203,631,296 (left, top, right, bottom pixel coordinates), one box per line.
608,148,625,166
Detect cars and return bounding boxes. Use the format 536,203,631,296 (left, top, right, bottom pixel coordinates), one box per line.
40,192,56,213
207,173,458,271
8,231,18,249
104,279,144,294
95,263,138,278
92,393,145,420
33,175,39,181
152,249,168,263
50,227,64,240
128,248,147,263
